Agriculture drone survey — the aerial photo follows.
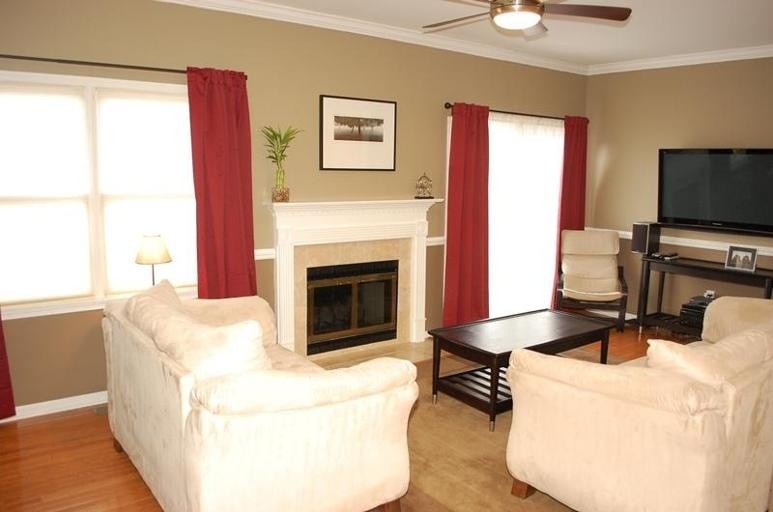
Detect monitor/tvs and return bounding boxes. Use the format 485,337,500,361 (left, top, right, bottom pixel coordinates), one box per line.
657,148,773,238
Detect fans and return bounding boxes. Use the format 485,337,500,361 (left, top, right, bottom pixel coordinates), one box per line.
422,0,632,37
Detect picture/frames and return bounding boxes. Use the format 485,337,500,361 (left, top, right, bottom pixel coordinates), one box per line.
319,94,397,171
725,244,758,272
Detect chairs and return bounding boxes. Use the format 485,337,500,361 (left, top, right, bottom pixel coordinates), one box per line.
558,229,628,333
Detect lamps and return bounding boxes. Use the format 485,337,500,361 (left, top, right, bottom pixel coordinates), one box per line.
490,5,544,30
136,235,172,286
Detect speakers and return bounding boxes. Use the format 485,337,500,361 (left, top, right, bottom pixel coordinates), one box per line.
629,222,660,254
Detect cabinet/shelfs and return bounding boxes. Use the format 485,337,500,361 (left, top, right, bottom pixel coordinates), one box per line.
638,257,773,336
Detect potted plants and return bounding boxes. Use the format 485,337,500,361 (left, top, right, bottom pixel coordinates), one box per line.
257,124,305,202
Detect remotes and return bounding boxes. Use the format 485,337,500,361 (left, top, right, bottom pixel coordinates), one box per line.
665,255,680,260
664,252,678,257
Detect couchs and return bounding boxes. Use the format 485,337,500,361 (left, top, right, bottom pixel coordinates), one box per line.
506,296,773,512
102,279,419,512
